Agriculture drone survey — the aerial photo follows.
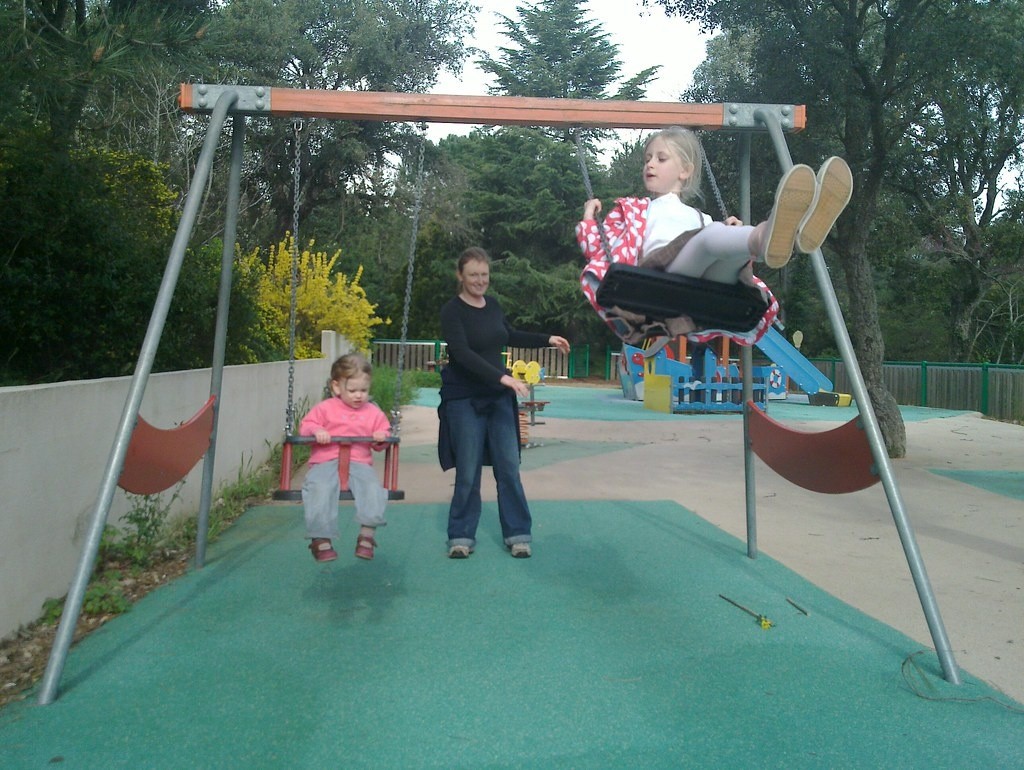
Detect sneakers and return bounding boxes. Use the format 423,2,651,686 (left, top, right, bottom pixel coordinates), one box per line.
449,545,473,557
507,543,532,557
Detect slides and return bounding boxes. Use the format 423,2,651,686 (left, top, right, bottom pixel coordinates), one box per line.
754,326,834,395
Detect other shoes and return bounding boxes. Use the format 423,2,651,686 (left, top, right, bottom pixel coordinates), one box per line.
308,537,336,561
764,164,816,270
356,536,377,559
796,155,853,254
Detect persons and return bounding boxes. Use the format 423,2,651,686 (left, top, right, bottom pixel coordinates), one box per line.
439,248,571,558
574,123,853,349
298,353,394,563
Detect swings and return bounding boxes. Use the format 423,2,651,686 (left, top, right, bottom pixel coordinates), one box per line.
269,118,430,504
574,126,776,336
